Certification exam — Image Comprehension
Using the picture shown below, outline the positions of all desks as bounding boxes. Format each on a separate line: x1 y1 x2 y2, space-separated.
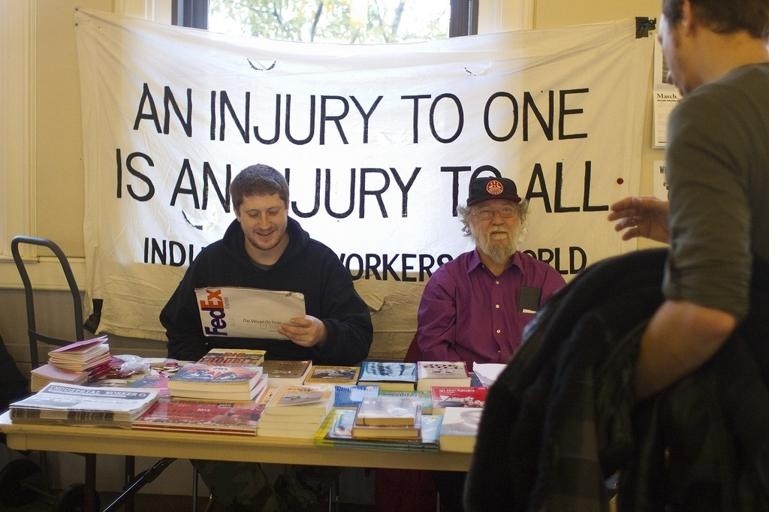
0 409 474 512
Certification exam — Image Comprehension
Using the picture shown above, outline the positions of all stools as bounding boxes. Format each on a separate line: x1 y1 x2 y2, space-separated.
193 467 340 512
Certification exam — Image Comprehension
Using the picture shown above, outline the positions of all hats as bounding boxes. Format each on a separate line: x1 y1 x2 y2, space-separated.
467 176 522 207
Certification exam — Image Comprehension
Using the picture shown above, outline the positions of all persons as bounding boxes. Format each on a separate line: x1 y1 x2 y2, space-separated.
608 0 766 512
158 165 373 511
407 174 571 511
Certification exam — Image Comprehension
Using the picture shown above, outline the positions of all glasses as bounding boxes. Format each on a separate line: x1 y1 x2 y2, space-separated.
477 207 516 221
